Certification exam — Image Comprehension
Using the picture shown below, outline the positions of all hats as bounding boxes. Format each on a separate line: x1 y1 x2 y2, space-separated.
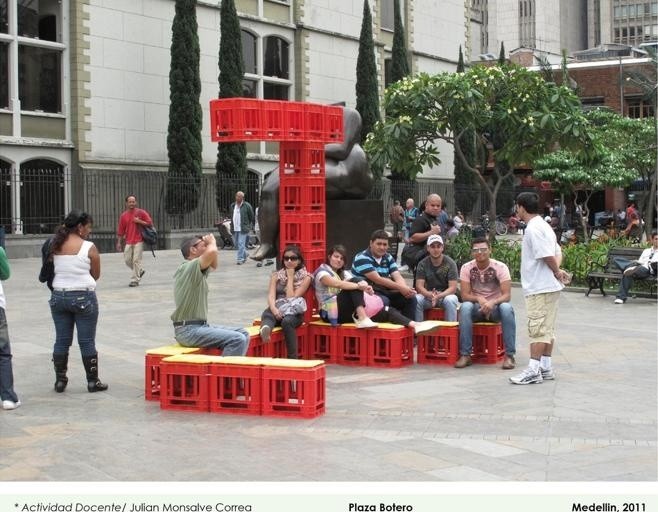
426 233 444 247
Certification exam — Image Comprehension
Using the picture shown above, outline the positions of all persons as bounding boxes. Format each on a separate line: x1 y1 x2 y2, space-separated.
352 230 418 321
543 199 566 227
259 246 311 359
508 192 570 384
390 201 405 239
613 232 658 304
509 205 526 233
39 210 108 392
313 245 441 335
452 211 464 227
117 196 153 288
255 206 274 268
599 204 647 240
231 191 254 265
171 234 250 356
455 238 515 369
404 198 418 240
410 194 441 292
435 203 450 225
415 235 458 322
442 220 459 242
0 247 22 411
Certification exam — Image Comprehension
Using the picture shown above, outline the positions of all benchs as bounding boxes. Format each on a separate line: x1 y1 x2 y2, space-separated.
585 246 658 301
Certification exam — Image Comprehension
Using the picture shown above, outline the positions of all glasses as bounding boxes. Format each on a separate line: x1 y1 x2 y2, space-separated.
282 255 301 261
471 246 490 254
649 253 655 259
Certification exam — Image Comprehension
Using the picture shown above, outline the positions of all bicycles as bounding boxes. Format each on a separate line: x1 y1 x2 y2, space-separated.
215 219 258 249
486 215 508 234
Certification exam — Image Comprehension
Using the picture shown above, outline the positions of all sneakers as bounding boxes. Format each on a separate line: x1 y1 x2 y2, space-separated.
414 319 441 338
355 316 380 330
613 296 626 306
237 259 247 265
260 324 272 344
256 262 263 267
244 253 249 258
128 280 139 288
622 262 641 278
1 398 22 409
542 368 557 382
264 261 275 266
139 267 145 278
508 370 545 386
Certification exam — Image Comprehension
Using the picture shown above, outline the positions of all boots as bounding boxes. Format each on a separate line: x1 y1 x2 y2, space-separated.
53 352 69 391
80 353 107 393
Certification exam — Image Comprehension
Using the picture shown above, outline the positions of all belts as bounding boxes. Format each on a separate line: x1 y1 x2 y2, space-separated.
173 320 207 326
54 287 96 291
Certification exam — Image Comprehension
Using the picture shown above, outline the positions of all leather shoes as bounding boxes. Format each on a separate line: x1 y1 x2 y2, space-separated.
502 355 515 370
453 353 475 367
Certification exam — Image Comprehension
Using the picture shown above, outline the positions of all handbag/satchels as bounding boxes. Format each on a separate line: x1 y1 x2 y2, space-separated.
401 215 435 275
275 297 308 318
138 208 159 246
